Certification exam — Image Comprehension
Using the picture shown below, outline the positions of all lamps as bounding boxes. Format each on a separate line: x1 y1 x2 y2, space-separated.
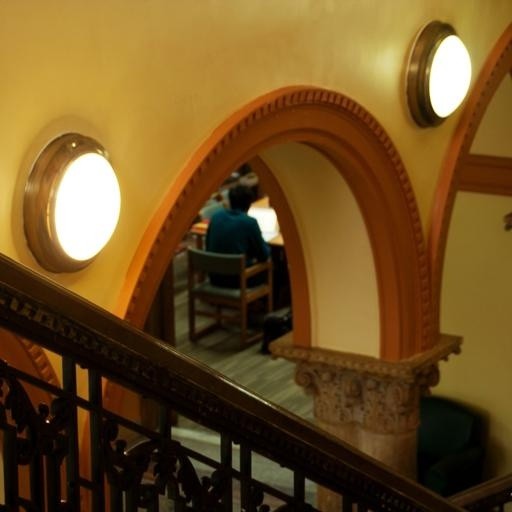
403 19 471 129
23 131 123 276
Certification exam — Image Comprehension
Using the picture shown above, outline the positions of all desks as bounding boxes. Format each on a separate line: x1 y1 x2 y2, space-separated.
191 195 287 251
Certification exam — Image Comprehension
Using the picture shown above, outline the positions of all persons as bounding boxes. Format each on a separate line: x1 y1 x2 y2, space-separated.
205 185 286 315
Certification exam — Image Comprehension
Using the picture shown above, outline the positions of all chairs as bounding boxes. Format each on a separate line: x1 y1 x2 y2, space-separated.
185 245 275 351
